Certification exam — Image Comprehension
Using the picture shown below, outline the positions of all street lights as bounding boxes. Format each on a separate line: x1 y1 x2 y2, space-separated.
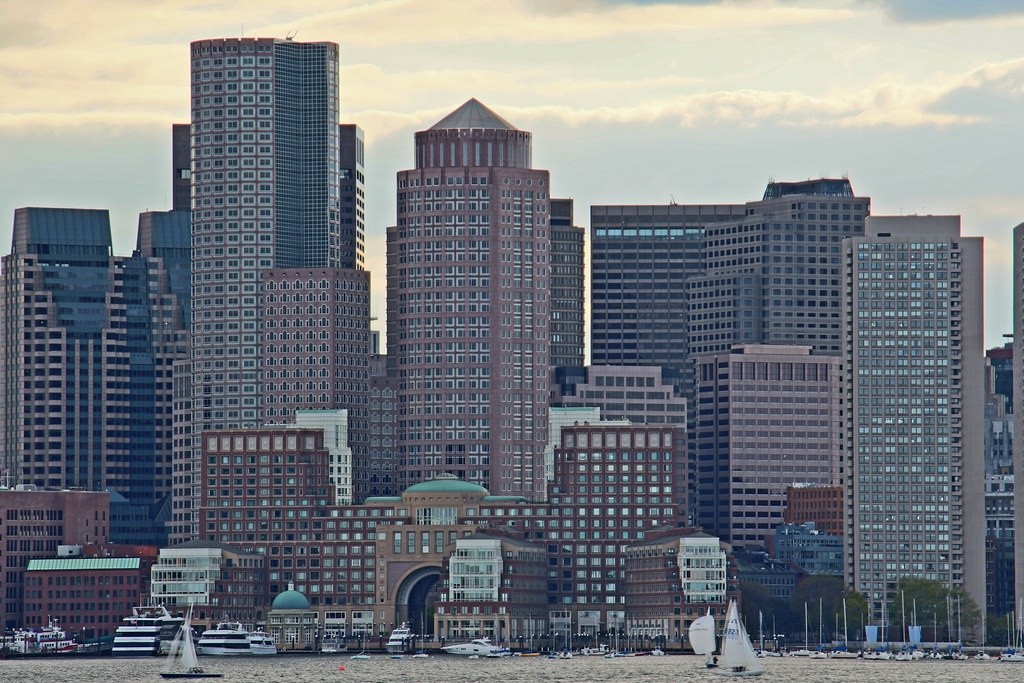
83 627 86 650
12 626 15 643
531 633 785 654
92 625 94 648
292 634 429 650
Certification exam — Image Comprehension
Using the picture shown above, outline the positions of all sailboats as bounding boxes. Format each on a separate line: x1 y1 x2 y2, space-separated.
912 595 1024 662
159 602 224 678
689 605 719 668
831 598 859 658
809 598 828 659
789 602 819 656
863 605 894 659
712 597 766 677
894 590 913 661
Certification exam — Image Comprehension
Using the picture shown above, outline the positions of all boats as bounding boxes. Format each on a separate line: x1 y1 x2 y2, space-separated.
196 622 277 655
490 647 513 656
386 622 415 653
112 606 170 656
350 655 371 660
412 654 428 658
441 639 500 656
520 650 540 656
560 651 572 658
584 648 605 655
